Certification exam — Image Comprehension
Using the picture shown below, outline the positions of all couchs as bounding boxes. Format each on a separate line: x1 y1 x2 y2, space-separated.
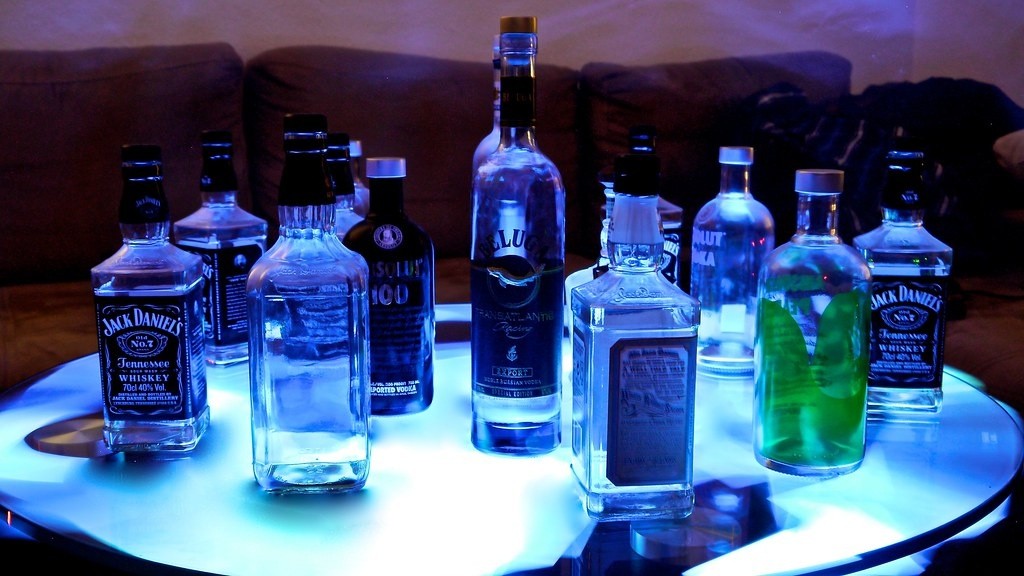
1 34 1023 411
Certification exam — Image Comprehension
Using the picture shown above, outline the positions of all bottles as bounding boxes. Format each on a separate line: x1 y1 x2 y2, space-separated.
612 131 686 292
91 142 212 454
246 112 372 495
347 138 371 218
472 33 510 199
691 146 775 377
320 132 366 248
571 149 701 522
853 150 954 417
467 15 563 457
171 129 269 368
341 156 434 419
563 180 631 450
753 168 868 477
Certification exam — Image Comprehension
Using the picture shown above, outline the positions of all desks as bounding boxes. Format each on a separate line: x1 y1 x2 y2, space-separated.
0 303 1023 575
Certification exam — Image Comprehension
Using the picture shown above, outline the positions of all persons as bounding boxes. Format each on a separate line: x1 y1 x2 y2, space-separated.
682 76 1023 311
